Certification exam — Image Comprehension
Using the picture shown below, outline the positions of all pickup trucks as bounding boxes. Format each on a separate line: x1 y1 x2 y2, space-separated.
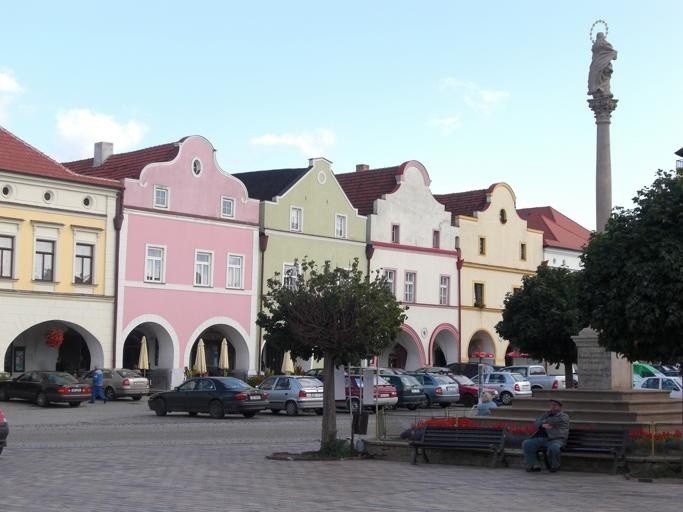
496 365 566 390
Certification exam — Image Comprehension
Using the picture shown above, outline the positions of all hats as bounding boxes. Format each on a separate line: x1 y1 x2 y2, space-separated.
549 400 562 406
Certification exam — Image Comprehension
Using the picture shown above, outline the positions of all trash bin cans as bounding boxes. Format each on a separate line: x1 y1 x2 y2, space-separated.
0 372 11 402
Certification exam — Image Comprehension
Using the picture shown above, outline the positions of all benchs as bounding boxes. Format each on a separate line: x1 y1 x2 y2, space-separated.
409 425 510 468
528 428 630 475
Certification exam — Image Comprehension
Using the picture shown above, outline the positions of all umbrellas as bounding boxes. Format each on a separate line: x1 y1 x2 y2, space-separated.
471 351 495 364
194 338 207 377
137 336 149 377
505 351 530 365
281 350 294 375
218 338 229 376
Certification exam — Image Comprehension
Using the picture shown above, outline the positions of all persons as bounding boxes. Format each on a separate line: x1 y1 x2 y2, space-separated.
523 399 570 473
89 365 106 403
133 365 142 374
475 393 498 416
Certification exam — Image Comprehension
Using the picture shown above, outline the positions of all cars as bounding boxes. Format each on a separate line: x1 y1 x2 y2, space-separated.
0 368 149 407
632 363 682 399
0 407 9 455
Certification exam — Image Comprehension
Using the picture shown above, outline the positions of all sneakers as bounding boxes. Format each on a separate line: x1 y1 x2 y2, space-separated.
550 466 560 472
526 467 541 472
